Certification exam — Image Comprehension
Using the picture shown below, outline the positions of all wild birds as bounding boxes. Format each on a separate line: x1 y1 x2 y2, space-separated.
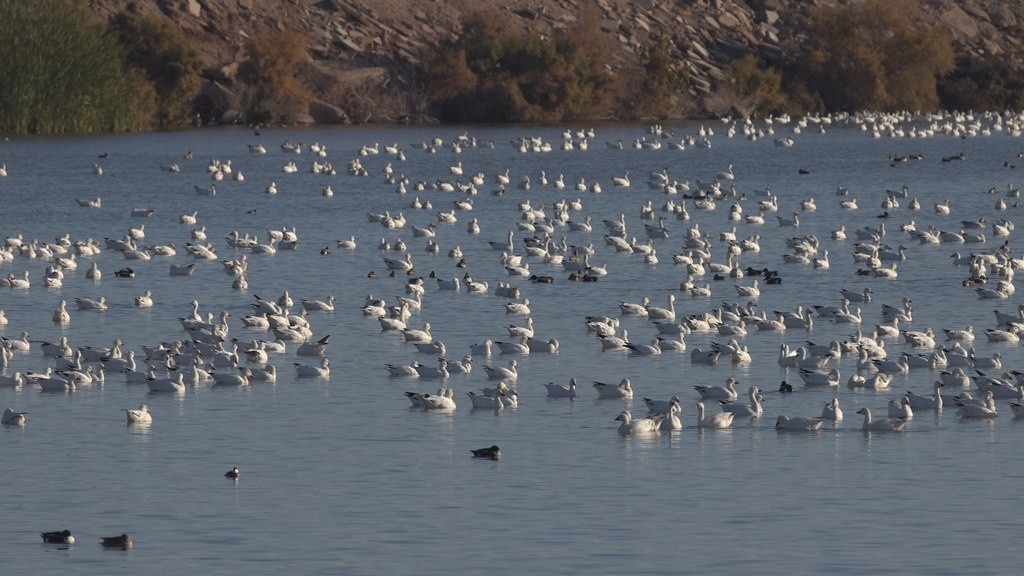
40 530 75 544
224 467 240 478
722 109 1022 146
587 121 1024 443
0 125 612 432
469 445 501 456
101 534 133 550
